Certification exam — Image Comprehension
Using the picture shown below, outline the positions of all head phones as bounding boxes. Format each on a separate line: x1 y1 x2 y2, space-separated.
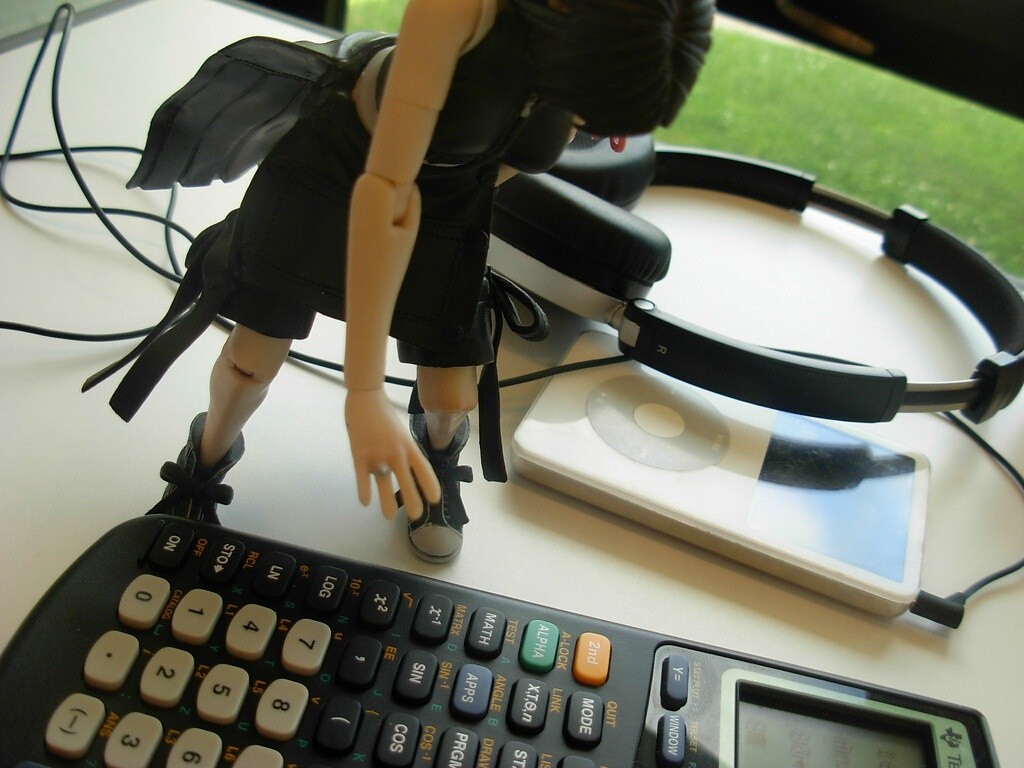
488 128 1024 425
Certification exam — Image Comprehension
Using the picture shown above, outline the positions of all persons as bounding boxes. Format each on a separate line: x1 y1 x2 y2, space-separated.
146 0 715 565
